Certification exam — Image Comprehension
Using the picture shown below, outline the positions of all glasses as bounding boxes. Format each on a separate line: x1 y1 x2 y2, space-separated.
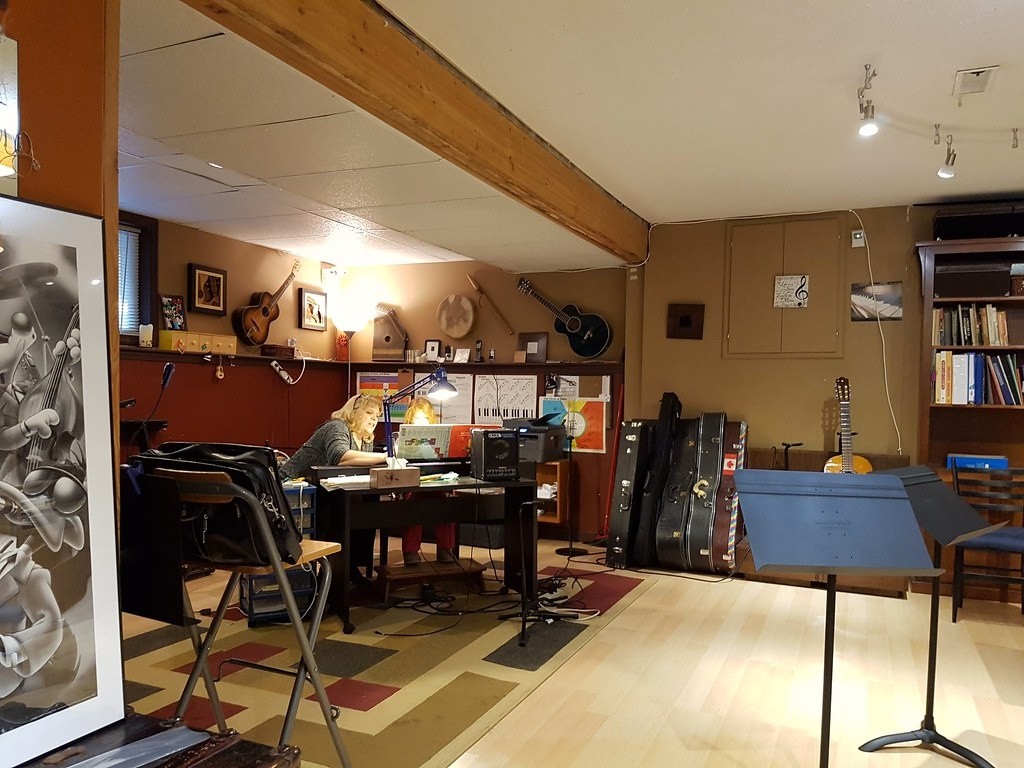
354 393 367 405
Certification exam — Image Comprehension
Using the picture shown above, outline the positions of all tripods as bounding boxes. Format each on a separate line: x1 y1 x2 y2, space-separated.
497 501 579 647
859 464 996 768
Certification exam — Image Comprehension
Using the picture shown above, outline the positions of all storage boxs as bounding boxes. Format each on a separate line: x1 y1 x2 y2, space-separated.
261 344 294 359
158 330 237 355
371 468 420 488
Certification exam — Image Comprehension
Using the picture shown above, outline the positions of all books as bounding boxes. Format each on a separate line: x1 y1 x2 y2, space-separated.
397 424 502 459
932 303 1008 346
932 349 1024 406
947 453 1008 471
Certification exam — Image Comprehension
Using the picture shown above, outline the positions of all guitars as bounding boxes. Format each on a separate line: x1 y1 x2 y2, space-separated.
230 258 303 347
516 276 614 359
822 374 874 476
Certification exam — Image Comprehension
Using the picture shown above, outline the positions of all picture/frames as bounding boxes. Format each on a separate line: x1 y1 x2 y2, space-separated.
298 288 327 331
187 263 227 316
0 193 125 768
159 293 188 330
424 339 441 362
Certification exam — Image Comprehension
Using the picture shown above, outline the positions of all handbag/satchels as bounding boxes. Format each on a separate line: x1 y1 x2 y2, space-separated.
142 441 303 565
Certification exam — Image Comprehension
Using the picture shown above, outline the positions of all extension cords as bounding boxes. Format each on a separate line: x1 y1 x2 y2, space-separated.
271 360 293 385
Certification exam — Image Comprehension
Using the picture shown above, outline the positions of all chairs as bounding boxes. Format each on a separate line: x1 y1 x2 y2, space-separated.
950 457 1024 623
133 466 355 768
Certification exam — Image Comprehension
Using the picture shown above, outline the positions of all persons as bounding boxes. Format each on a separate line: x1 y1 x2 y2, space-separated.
393 397 455 564
278 392 388 588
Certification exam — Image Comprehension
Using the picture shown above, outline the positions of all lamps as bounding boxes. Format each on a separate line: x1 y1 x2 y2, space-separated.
382 364 458 457
330 298 367 400
860 100 879 137
937 135 956 178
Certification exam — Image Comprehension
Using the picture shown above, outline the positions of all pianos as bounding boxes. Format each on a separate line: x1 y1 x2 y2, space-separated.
309 460 541 633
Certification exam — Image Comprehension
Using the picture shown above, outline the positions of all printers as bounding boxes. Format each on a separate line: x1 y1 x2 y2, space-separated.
503 413 566 464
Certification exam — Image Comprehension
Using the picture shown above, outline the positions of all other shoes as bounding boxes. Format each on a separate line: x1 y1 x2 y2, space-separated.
404 551 420 565
350 568 373 587
437 548 454 562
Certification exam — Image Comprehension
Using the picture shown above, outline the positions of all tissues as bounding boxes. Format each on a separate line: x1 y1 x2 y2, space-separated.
370 457 420 489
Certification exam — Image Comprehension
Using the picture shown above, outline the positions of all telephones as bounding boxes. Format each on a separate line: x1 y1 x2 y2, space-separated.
473 339 484 363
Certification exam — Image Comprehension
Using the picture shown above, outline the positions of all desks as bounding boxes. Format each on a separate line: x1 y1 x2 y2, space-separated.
302 472 538 635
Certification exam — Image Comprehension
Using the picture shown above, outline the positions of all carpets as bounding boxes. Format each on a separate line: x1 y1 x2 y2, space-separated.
123 549 659 768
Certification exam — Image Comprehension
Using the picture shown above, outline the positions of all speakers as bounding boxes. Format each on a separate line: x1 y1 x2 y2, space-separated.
472 428 520 480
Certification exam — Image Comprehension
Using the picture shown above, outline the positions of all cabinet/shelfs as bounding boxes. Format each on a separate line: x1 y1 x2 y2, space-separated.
910 238 1024 602
723 213 843 358
239 483 317 628
